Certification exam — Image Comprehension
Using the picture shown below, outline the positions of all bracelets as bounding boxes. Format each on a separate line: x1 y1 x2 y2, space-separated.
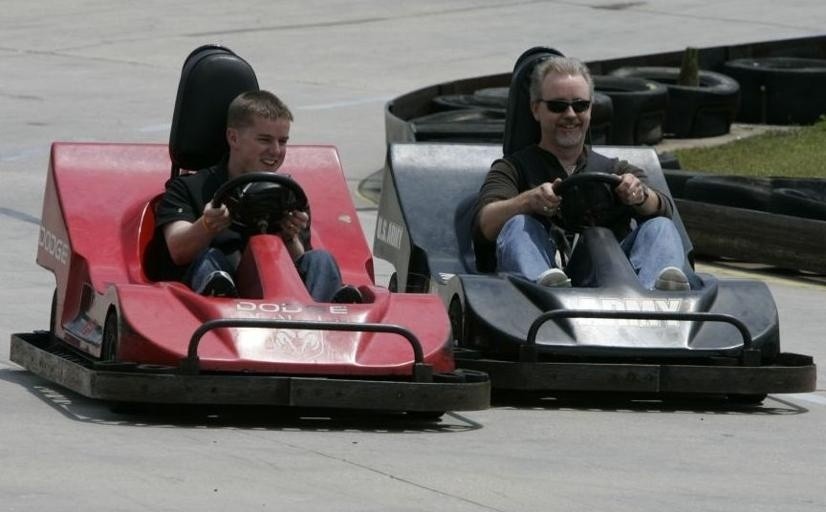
634 184 650 206
200 215 215 236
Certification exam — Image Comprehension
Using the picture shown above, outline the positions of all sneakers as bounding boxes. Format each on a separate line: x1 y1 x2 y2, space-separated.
655 267 690 292
198 271 236 297
537 268 571 287
331 286 363 303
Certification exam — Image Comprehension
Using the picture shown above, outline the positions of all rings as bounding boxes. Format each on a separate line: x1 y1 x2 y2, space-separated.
542 205 549 215
286 224 294 229
630 191 638 197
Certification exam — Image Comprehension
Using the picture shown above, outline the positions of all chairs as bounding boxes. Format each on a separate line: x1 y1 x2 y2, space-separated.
454 45 567 273
137 45 261 280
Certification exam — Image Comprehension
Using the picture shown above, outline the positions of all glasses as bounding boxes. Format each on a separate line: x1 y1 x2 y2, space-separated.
537 99 591 113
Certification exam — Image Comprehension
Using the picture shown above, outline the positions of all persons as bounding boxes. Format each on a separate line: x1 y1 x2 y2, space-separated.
469 54 704 296
141 88 367 306
